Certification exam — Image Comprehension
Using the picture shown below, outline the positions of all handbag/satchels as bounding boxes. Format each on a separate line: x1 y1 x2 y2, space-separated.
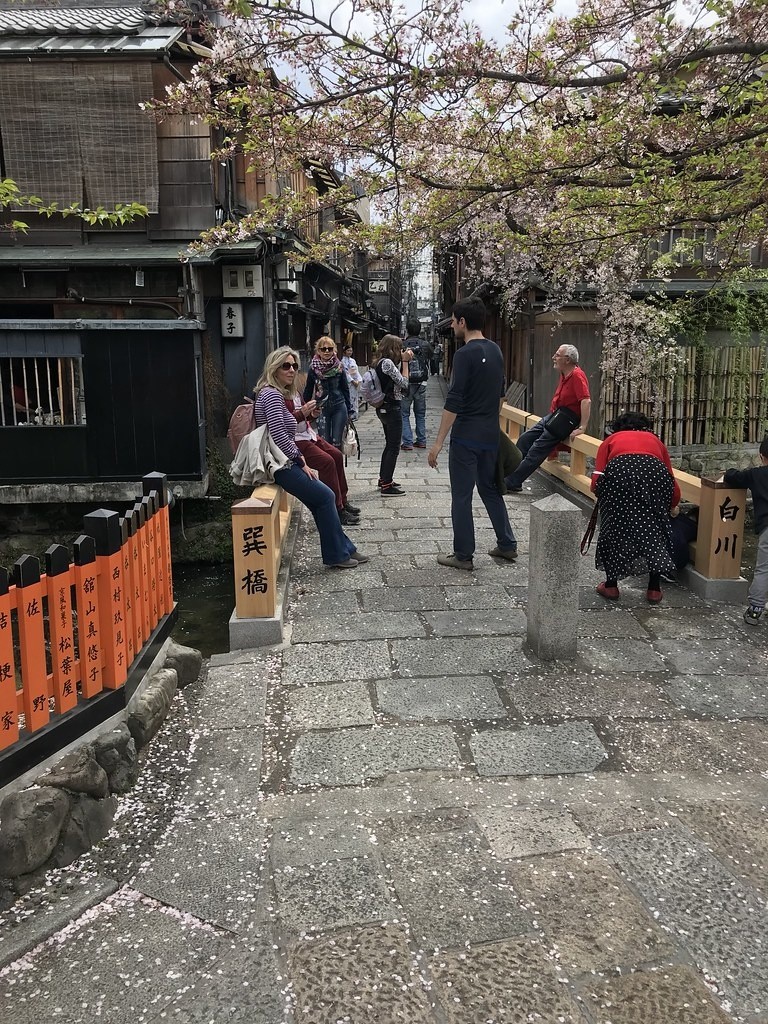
544 407 580 440
341 418 359 456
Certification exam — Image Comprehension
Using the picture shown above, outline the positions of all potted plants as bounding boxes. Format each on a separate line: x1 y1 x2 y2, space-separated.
371 337 378 352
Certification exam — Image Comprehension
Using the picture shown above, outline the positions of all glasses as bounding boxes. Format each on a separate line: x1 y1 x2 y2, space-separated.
318 346 334 352
554 353 569 358
277 362 299 371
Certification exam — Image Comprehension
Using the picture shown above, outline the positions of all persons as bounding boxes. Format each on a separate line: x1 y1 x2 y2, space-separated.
400 319 435 450
723 435 768 626
428 296 518 568
497 344 591 494
400 335 443 376
590 412 681 603
371 334 414 497
254 345 369 568
341 345 363 422
303 337 352 451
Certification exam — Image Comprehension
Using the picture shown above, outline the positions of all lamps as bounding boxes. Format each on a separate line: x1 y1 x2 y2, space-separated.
365 298 373 308
384 314 389 321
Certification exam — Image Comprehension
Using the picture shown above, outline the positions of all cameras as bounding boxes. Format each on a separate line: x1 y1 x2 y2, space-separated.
403 345 421 355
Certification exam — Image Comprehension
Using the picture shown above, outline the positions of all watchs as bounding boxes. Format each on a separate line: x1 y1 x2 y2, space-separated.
579 426 586 431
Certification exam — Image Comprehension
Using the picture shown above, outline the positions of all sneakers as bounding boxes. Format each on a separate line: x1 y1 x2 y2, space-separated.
378 478 402 487
660 571 676 582
596 582 620 599
437 553 474 569
338 501 361 525
328 551 369 568
381 486 406 496
647 589 663 603
743 606 763 625
487 546 519 559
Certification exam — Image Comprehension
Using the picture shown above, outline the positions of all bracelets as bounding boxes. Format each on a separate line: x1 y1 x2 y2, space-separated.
402 361 408 362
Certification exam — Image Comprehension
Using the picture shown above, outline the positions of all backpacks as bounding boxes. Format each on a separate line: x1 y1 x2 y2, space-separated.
359 358 386 408
403 340 430 382
226 403 257 457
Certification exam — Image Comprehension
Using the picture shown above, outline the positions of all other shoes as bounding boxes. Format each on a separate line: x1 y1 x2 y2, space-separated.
503 480 523 492
401 443 414 450
413 441 427 448
